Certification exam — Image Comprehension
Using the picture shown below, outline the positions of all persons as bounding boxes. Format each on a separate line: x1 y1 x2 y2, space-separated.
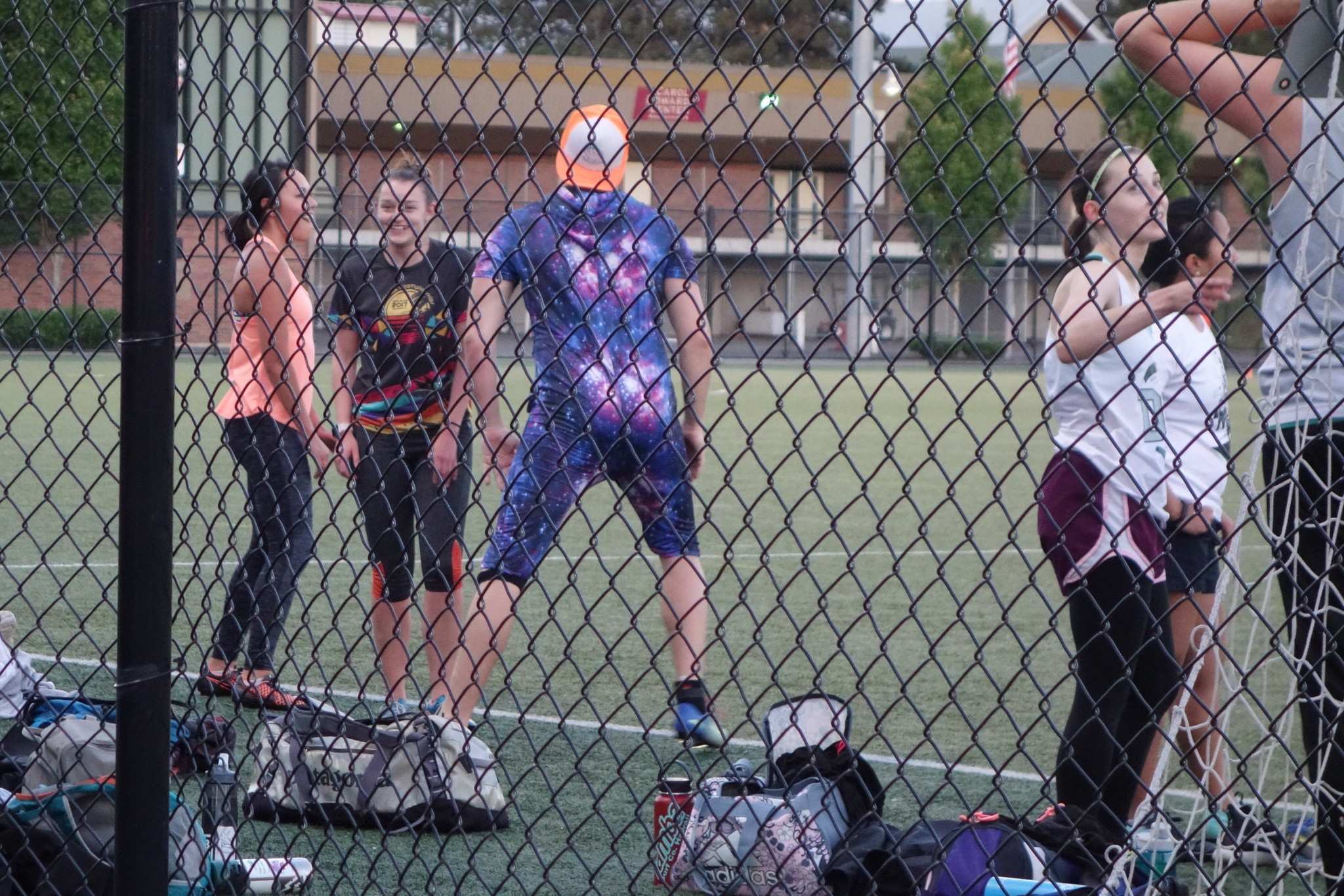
1110 0 1343 896
325 160 480 732
442 102 734 752
1037 139 1233 896
1121 195 1234 845
196 161 342 711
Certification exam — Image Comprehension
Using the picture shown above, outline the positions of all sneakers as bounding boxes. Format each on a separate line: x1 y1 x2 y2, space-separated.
672 702 730 749
1125 811 1217 860
196 664 246 695
1211 802 1281 866
1276 813 1324 870
1201 803 1231 843
232 675 308 710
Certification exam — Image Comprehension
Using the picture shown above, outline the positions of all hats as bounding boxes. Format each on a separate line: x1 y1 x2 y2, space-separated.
555 104 631 193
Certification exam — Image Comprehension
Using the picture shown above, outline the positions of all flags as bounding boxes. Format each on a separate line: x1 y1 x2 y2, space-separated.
1004 4 1023 86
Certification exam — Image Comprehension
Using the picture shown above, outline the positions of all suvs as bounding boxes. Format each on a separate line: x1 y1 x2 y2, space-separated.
815 300 896 342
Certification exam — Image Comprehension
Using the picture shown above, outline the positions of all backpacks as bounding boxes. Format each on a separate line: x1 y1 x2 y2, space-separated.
21 711 116 793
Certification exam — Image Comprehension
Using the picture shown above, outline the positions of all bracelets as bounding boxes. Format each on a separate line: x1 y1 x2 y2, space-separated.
336 423 355 434
1171 497 1187 524
450 421 461 429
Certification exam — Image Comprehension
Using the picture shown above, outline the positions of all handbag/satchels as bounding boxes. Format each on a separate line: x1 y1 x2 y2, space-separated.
668 773 849 896
241 700 511 834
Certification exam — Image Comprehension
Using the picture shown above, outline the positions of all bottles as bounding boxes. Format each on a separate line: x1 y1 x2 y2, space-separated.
652 759 698 884
209 857 314 895
201 752 241 862
1133 819 1178 883
